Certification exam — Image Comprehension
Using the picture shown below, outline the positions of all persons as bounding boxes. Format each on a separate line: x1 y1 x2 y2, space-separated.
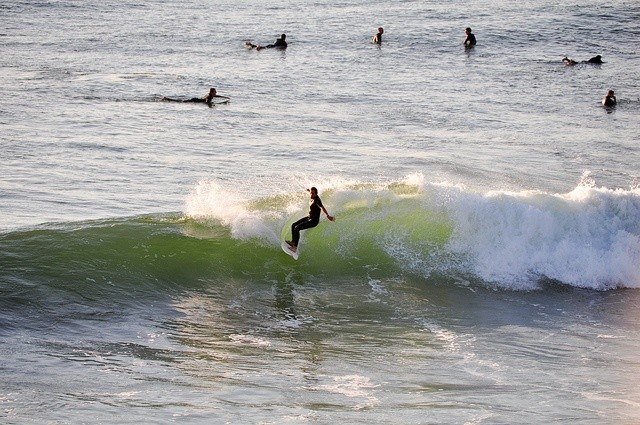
372 29 384 46
285 185 337 253
463 27 477 51
244 33 289 52
160 88 233 108
601 89 617 113
562 55 605 65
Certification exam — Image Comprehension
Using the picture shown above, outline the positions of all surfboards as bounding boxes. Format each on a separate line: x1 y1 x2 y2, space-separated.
281 222 300 260
216 98 229 104
